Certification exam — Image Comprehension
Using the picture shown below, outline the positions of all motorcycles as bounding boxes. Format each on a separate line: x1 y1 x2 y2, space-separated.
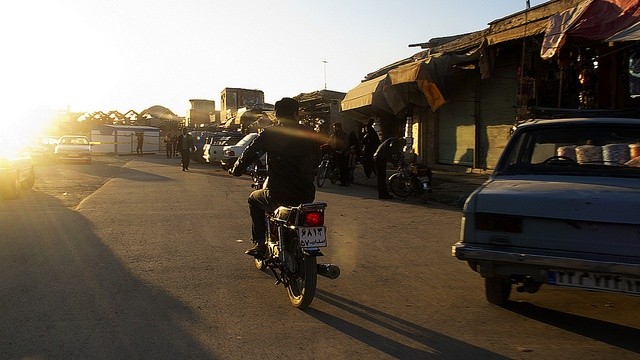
230 165 340 311
317 146 354 187
389 150 433 204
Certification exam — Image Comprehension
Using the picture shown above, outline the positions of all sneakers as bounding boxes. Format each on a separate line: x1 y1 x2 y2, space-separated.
244 244 269 261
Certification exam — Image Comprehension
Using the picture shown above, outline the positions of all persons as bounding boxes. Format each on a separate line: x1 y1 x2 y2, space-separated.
176 133 183 152
135 134 144 156
319 121 351 186
360 123 379 179
228 97 322 256
171 134 180 157
372 137 408 199
164 132 175 159
175 128 195 171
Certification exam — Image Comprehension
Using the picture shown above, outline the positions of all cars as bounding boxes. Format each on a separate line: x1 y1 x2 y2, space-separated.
187 131 223 166
450 118 638 306
223 131 266 177
224 132 243 146
54 136 92 165
